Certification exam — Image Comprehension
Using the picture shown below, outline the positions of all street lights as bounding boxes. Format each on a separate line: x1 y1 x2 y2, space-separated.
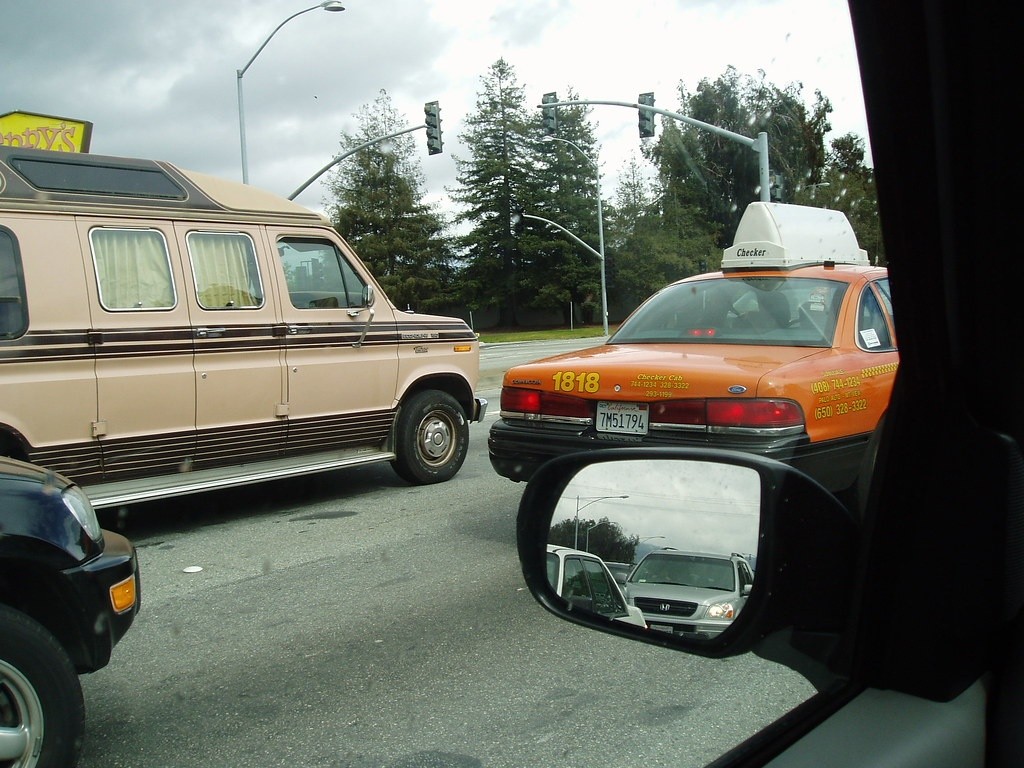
236 0 346 187
586 522 619 551
520 136 610 337
634 535 666 565
574 494 629 552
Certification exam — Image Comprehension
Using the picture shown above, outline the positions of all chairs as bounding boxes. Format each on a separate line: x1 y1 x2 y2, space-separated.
798 300 828 328
733 311 770 331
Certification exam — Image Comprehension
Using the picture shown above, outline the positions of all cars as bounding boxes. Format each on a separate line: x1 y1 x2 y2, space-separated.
624 547 755 640
488 200 900 505
547 543 648 628
0 455 142 768
605 562 639 605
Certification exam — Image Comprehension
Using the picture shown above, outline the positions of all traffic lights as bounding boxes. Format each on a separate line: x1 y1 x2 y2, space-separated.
638 91 657 138
542 92 559 134
424 101 445 156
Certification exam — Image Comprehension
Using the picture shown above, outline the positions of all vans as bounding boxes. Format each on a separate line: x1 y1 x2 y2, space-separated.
0 145 489 511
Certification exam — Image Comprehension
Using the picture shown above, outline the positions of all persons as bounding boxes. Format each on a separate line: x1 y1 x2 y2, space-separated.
757 291 792 327
